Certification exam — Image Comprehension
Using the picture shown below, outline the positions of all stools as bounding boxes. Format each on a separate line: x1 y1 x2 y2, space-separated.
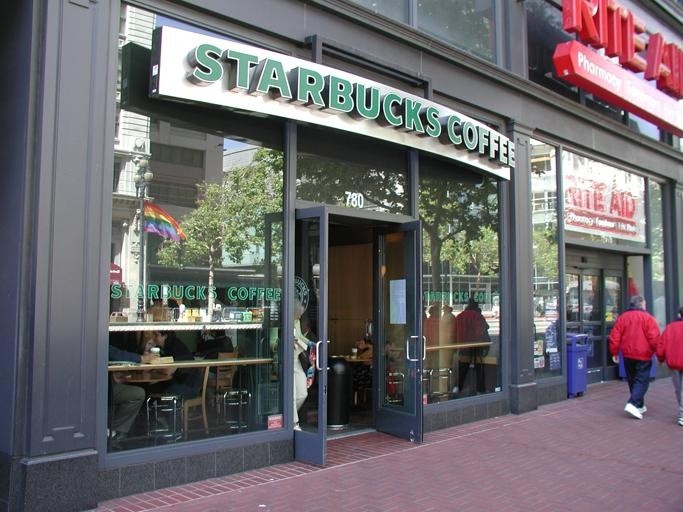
386 369 406 408
146 391 184 446
420 368 453 406
216 388 250 433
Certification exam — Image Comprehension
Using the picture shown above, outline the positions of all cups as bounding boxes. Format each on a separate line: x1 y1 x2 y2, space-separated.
150 347 160 358
351 347 358 359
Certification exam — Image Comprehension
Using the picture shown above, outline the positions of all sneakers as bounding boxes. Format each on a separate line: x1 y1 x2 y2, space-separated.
624 403 646 419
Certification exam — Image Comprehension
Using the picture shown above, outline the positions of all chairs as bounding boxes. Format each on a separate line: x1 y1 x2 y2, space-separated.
209 345 242 413
178 362 212 433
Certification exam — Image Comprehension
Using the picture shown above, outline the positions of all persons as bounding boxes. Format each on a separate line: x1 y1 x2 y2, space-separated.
196 330 234 380
273 296 375 433
422 299 492 402
145 330 202 442
657 306 683 426
108 344 159 451
608 296 660 420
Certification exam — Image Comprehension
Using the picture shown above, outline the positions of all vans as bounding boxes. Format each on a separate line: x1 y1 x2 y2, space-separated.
566 279 620 321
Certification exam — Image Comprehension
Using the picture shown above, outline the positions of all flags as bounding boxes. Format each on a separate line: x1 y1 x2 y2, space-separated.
142 202 185 241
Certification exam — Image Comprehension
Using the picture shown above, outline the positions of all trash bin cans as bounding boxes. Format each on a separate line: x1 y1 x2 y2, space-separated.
618 350 658 381
566 333 592 399
327 357 352 431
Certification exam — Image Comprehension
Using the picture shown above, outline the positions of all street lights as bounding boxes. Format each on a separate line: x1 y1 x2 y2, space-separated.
130 158 155 322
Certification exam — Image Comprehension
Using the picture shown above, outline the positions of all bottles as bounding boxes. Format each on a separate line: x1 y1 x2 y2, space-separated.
177 303 186 322
